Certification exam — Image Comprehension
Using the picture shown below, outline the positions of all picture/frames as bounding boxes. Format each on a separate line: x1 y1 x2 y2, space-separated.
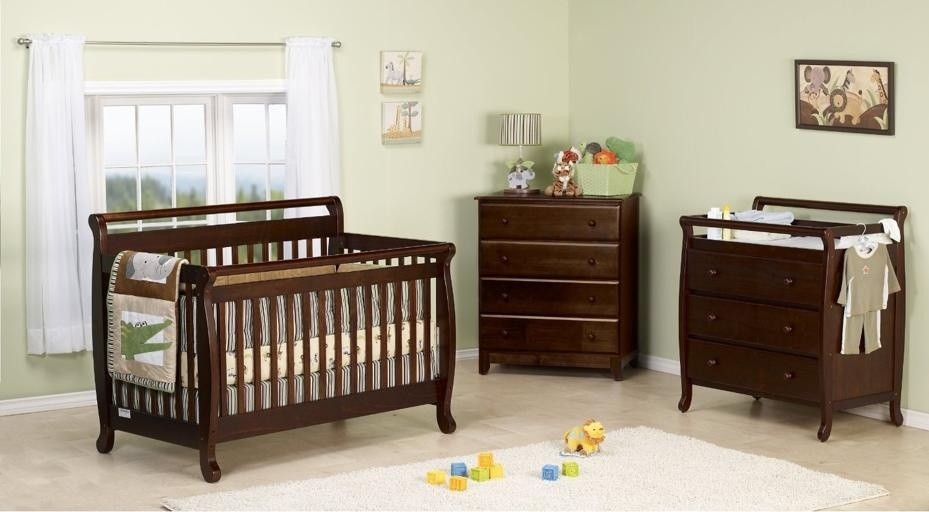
379 48 426 148
791 58 898 138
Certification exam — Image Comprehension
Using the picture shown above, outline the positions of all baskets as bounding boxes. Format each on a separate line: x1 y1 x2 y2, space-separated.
575 163 639 197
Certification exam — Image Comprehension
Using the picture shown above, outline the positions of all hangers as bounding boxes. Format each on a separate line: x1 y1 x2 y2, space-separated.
850 219 877 254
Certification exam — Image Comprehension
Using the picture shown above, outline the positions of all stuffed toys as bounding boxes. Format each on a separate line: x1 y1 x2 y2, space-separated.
545 136 637 197
563 416 606 455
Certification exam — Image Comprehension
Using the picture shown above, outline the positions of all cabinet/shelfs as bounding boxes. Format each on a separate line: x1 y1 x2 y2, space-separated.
471 187 646 384
676 192 913 445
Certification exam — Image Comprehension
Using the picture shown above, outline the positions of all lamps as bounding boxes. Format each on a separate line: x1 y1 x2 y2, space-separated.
498 112 544 196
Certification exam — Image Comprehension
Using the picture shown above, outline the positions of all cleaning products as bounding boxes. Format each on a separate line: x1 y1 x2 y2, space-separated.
706 208 722 240
723 204 732 240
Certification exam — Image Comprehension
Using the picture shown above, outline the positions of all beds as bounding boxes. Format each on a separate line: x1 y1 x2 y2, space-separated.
84 196 462 485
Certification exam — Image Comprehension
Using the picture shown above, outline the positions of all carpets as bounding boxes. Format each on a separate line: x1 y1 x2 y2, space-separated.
153 424 899 508
